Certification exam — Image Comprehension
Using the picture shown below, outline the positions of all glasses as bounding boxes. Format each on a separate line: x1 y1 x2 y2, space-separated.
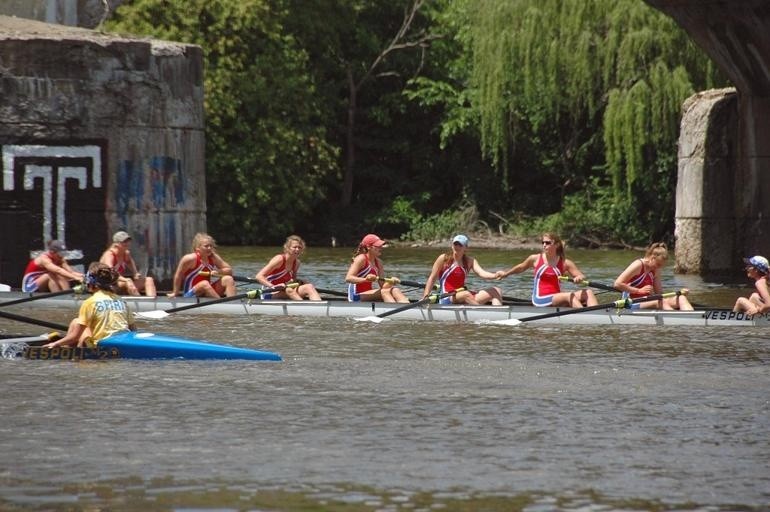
542 241 553 244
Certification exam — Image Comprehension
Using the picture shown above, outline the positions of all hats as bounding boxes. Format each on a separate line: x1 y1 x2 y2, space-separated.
453 235 468 246
362 234 385 248
744 256 769 273
50 240 68 257
112 231 130 244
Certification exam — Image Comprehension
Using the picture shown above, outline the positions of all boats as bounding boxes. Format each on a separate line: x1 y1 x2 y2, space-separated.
0 287 770 328
0 331 284 362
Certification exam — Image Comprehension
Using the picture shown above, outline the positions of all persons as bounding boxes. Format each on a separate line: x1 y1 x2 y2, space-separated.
255 235 322 301
99 230 157 296
167 232 236 298
733 255 770 316
613 242 694 311
424 234 503 306
345 234 410 303
22 240 84 293
496 232 598 308
41 261 137 349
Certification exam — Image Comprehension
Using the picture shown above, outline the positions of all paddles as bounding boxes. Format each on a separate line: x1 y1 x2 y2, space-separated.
0 283 22 291
137 283 299 319
354 286 467 324
491 289 687 327
